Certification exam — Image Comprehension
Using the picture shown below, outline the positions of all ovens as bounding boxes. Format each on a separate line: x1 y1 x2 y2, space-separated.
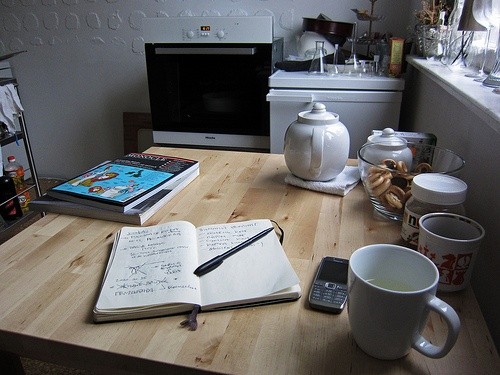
141 14 284 151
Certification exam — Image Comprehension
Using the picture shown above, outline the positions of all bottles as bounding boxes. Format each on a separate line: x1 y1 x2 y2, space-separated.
308 41 329 76
0 175 23 226
4 155 32 211
401 173 468 250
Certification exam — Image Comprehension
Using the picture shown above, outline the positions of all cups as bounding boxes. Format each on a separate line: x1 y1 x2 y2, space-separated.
347 244 460 361
418 213 486 293
305 48 327 72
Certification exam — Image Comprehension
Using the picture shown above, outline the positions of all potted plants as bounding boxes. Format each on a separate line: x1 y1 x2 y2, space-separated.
407 0 453 62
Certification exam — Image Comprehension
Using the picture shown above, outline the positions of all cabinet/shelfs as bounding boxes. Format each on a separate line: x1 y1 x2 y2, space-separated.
0 60 46 237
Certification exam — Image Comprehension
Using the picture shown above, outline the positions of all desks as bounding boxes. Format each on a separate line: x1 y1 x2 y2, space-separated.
0 147 500 375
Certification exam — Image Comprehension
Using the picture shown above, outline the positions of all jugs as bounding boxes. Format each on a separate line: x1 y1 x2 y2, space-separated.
283 103 350 183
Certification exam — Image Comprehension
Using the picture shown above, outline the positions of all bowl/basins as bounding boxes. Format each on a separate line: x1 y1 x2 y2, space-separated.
302 17 353 48
357 141 466 222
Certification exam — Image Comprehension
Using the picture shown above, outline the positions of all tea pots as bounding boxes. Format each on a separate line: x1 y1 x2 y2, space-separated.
295 30 334 57
364 128 413 183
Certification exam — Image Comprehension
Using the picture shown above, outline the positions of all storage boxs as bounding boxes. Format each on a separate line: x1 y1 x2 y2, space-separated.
386 37 403 77
372 130 437 168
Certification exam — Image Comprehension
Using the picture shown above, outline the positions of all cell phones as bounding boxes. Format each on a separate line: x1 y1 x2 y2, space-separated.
308 255 351 313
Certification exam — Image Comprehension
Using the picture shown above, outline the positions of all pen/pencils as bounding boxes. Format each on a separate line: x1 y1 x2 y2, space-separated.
194 227 274 277
